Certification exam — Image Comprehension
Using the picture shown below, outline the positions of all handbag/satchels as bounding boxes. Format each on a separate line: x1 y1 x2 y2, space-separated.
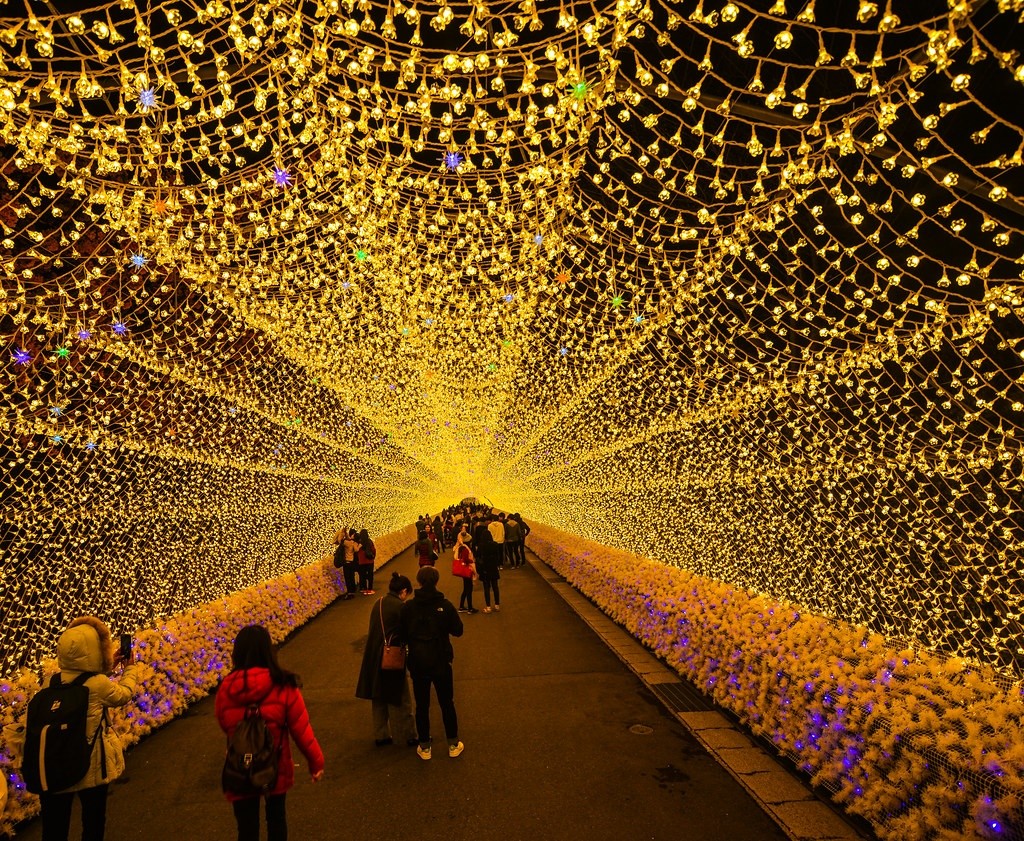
366 550 375 560
382 632 407 669
452 559 475 579
428 550 439 560
333 539 346 568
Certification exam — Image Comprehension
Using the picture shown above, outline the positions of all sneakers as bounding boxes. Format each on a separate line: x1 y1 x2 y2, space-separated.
448 741 464 757
417 745 432 760
468 608 478 614
458 607 468 612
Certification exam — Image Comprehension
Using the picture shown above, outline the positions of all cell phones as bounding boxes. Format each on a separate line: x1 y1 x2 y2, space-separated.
120 634 132 659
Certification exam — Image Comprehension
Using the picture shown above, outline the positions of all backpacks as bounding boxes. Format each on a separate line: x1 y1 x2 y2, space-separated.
221 685 288 798
22 671 111 795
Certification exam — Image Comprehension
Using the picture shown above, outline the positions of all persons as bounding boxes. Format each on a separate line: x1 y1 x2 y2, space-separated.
400 566 465 760
38 616 137 841
355 572 433 744
215 624 326 840
414 498 530 615
343 528 376 597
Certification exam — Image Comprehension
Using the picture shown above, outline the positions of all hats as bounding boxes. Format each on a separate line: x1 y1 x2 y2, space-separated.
458 531 472 542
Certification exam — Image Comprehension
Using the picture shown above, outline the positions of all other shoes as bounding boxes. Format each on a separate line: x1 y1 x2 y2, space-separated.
364 590 375 595
406 736 433 746
482 607 492 613
359 590 363 593
344 593 356 600
376 737 392 746
497 562 526 570
495 605 499 611
